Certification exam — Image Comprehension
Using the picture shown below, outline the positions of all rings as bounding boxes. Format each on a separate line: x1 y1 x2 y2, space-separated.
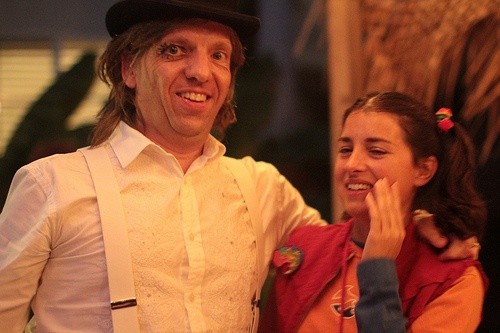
470 242 482 251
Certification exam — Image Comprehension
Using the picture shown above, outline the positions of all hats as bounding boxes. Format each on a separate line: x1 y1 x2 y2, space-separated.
105 0 262 45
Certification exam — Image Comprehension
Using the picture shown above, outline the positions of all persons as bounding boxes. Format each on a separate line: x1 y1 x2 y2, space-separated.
257 91 489 333
0 0 480 333
